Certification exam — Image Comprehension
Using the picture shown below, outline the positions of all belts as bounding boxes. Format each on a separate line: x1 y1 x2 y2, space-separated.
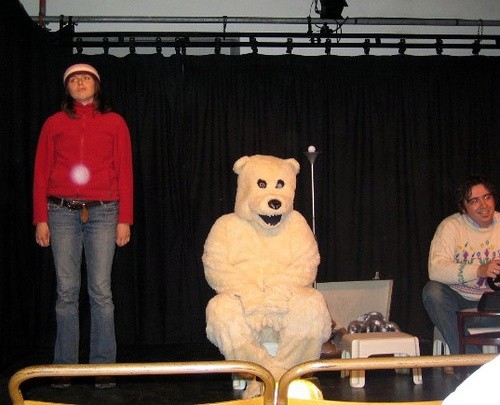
47 196 112 210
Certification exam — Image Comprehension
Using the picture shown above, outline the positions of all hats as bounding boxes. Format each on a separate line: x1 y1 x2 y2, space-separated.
63 63 101 83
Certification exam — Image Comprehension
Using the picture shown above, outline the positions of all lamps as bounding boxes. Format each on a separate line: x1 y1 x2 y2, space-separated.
303 146 321 289
313 0 349 19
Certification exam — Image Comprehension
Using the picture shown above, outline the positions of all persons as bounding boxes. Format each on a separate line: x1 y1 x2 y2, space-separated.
423 183 500 370
36 60 135 388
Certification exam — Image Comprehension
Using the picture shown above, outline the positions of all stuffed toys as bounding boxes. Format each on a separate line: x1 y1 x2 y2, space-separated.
201 152 331 398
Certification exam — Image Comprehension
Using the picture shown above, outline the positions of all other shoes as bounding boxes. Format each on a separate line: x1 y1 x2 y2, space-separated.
95 376 117 389
51 377 71 389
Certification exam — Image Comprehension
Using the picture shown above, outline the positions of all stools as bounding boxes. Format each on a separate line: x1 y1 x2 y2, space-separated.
341 332 422 387
433 307 500 369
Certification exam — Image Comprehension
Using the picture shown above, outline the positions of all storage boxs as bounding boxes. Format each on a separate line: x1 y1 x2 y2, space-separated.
315 277 396 379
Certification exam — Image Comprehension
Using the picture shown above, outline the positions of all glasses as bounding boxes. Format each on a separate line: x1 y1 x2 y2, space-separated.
467 195 491 205
70 76 91 84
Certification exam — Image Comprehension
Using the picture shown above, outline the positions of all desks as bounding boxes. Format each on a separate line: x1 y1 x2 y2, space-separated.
457 311 500 381
